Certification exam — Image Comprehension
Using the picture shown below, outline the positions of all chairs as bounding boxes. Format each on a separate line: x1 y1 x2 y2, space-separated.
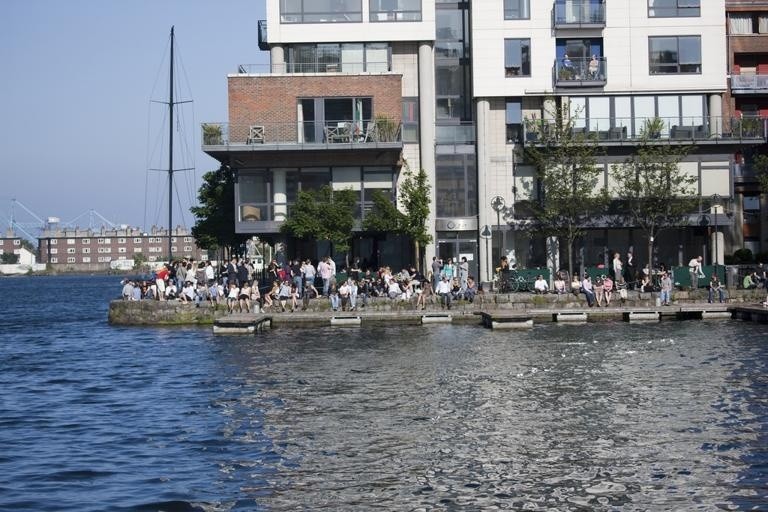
246 124 266 146
319 122 378 144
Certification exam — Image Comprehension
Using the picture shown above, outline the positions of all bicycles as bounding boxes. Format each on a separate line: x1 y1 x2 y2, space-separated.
501 271 539 293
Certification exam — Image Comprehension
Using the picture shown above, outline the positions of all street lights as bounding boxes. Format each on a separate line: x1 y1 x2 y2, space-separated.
491 196 509 270
708 193 722 282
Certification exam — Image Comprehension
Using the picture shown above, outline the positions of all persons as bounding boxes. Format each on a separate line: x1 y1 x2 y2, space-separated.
562 54 580 81
589 54 598 75
